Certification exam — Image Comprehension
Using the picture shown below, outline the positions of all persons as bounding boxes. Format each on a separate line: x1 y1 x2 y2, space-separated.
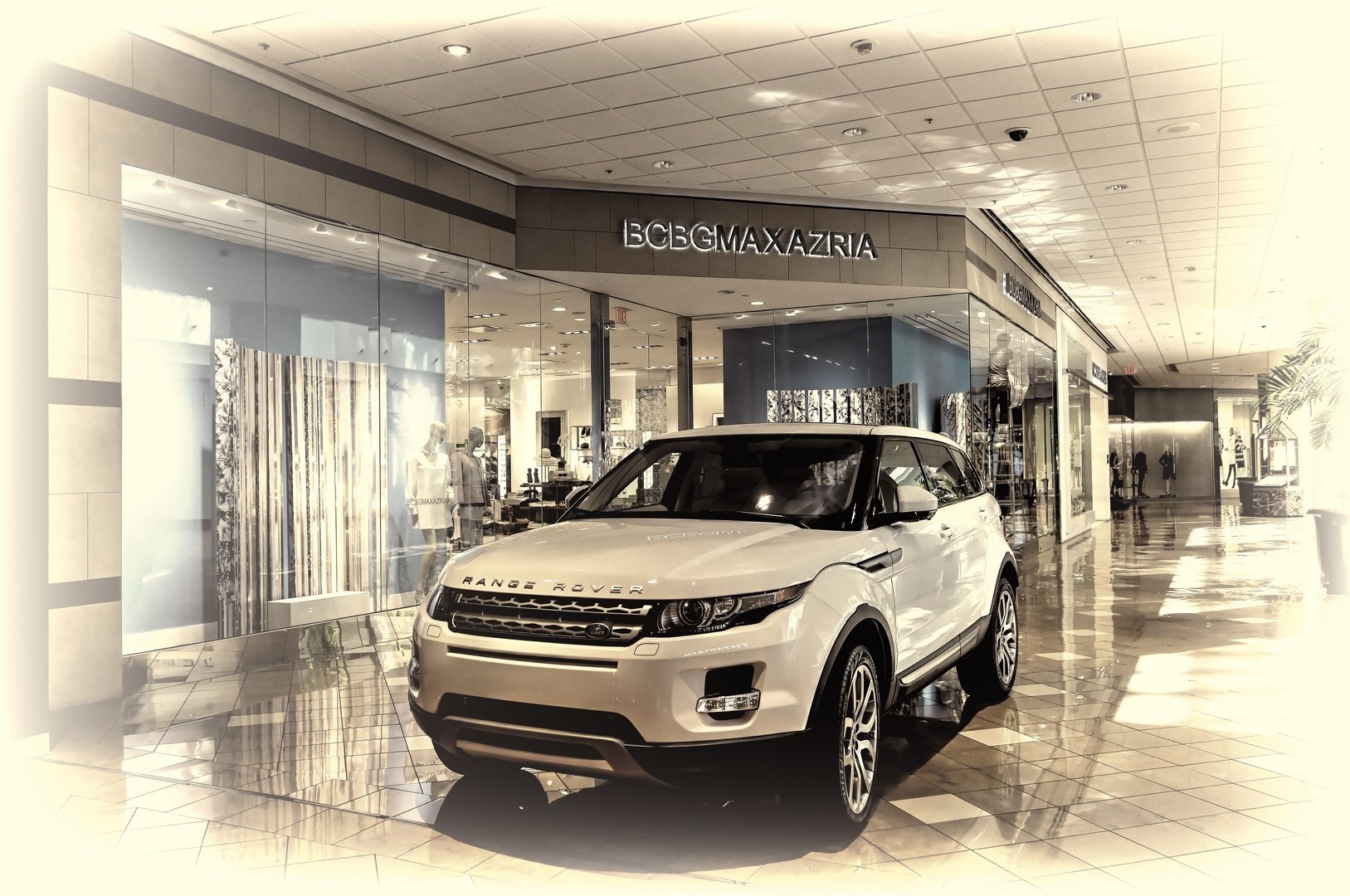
479 444 499 498
451 426 490 548
1233 428 1248 486
1221 426 1238 488
1134 446 1148 497
410 423 459 601
988 332 1012 439
1158 445 1176 495
1109 445 1120 498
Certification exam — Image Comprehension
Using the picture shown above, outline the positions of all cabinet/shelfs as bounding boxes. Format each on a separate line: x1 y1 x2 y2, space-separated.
523 479 593 531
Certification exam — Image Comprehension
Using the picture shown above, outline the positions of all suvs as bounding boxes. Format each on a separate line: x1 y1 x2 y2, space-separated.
404 421 1022 842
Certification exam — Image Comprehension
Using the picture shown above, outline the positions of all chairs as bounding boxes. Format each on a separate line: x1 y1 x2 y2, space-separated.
690 467 759 513
